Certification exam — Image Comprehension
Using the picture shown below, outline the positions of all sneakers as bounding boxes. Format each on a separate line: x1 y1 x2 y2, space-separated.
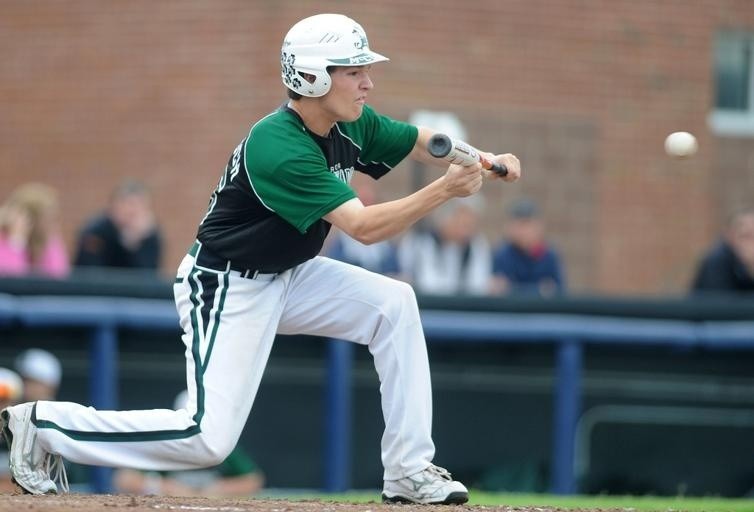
381 467 469 504
1 399 57 494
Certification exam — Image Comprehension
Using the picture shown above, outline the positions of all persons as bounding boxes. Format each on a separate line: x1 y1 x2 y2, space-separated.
0 10 523 505
392 186 513 299
686 204 754 295
75 183 164 270
494 199 569 298
0 347 95 493
109 387 264 498
0 366 26 480
320 173 415 290
0 181 72 281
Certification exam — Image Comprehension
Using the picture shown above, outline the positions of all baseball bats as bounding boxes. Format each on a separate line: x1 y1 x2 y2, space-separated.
427 134 506 174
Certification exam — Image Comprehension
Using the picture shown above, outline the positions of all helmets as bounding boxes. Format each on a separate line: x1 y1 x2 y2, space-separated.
280 14 390 98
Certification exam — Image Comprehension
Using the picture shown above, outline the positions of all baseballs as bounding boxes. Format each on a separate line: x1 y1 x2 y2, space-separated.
664 131 698 159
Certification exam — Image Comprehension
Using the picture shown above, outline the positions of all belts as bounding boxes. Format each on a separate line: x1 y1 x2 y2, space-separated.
188 242 277 275
